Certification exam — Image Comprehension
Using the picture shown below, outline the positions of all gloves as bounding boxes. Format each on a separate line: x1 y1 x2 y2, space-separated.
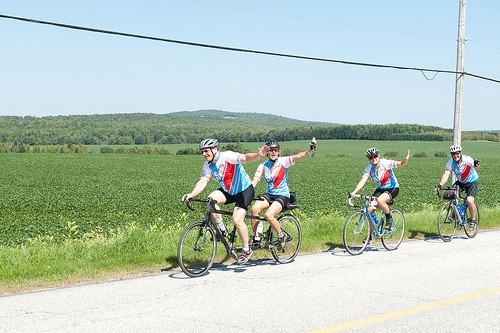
310 137 318 152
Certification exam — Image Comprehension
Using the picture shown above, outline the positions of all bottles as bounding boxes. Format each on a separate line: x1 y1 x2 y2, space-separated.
457 206 462 216
370 210 379 224
461 205 465 217
216 219 229 237
254 221 263 242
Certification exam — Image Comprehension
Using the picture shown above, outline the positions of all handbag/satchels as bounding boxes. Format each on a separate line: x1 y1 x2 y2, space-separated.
284 192 297 210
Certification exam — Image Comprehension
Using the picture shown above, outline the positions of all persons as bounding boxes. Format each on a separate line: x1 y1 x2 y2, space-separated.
182 138 270 264
248 137 317 251
348 147 410 244
434 144 481 231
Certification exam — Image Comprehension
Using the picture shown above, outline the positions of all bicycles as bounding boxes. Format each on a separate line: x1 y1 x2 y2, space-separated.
342 191 406 256
433 183 480 242
176 189 302 278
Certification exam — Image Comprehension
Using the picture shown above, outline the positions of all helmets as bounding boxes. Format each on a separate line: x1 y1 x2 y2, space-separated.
449 144 462 152
366 147 379 157
198 139 219 149
265 139 280 146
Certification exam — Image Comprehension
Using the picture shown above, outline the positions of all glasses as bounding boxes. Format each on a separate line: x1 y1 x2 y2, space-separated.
269 148 279 152
452 152 460 155
201 150 210 154
368 154 378 160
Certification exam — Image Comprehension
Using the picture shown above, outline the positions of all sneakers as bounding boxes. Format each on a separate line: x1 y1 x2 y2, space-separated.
277 233 287 251
469 219 477 230
364 236 372 244
238 248 253 265
385 216 393 229
211 229 228 241
